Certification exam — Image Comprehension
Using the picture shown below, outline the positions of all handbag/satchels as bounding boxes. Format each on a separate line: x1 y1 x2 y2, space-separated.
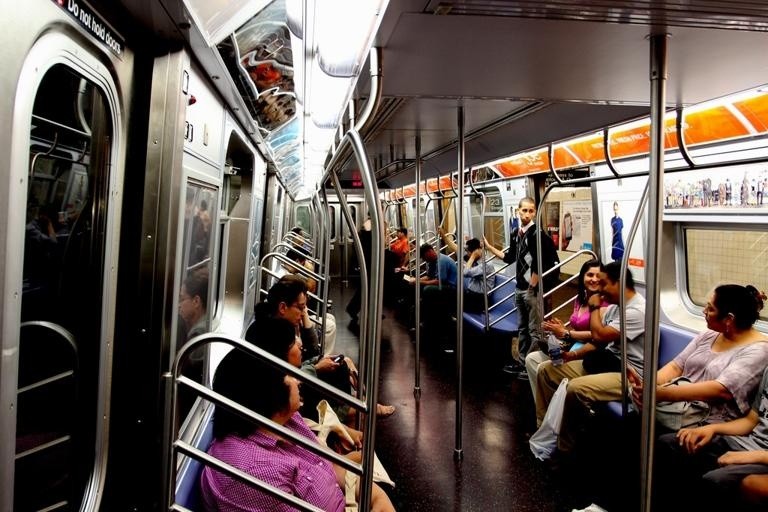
582 348 621 375
301 399 396 488
654 376 713 432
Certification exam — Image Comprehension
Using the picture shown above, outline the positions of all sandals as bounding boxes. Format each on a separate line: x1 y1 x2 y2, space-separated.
377 404 396 417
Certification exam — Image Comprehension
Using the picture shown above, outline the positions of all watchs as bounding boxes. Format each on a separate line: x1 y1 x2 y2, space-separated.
564 330 571 339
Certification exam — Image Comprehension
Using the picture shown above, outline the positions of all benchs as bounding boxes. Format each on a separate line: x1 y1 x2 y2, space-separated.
460 271 523 334
608 321 698 419
171 404 218 511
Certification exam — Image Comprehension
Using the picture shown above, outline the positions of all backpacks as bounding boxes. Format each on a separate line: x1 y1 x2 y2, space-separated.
547 236 561 280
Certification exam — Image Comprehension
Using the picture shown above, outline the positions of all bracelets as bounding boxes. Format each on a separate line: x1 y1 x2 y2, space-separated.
573 351 577 358
589 305 599 312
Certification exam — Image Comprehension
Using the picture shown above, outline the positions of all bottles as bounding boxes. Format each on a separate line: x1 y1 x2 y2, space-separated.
548 331 563 366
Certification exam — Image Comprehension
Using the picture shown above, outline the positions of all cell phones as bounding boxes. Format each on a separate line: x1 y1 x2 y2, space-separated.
333 354 344 362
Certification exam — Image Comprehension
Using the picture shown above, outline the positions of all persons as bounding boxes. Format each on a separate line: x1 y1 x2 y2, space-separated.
610 202 624 258
741 474 768 503
509 206 521 233
523 263 660 464
179 187 212 369
525 260 604 408
25 202 58 289
479 196 560 380
572 285 768 512
676 366 768 512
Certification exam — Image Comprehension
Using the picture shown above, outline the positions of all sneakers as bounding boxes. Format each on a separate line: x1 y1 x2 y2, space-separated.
518 370 529 381
345 307 359 321
571 503 609 512
503 362 523 374
